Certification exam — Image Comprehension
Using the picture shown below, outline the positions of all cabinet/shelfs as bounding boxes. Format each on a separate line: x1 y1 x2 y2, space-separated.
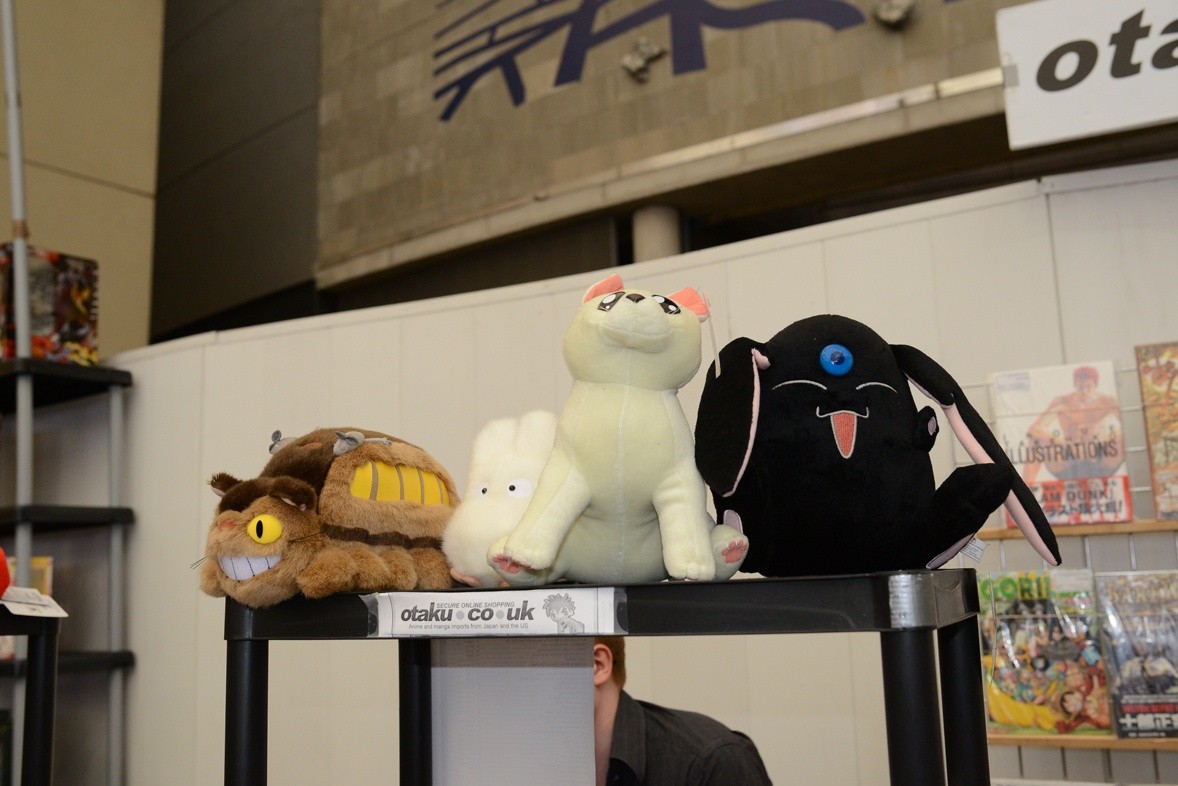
0 351 133 786
973 521 1178 753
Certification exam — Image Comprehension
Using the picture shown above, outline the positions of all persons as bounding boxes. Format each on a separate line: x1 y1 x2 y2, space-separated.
1020 362 1126 488
594 637 778 786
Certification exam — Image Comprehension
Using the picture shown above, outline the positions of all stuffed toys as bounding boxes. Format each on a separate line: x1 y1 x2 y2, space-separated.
694 312 1066 583
204 428 469 612
482 269 750 590
440 410 567 596
